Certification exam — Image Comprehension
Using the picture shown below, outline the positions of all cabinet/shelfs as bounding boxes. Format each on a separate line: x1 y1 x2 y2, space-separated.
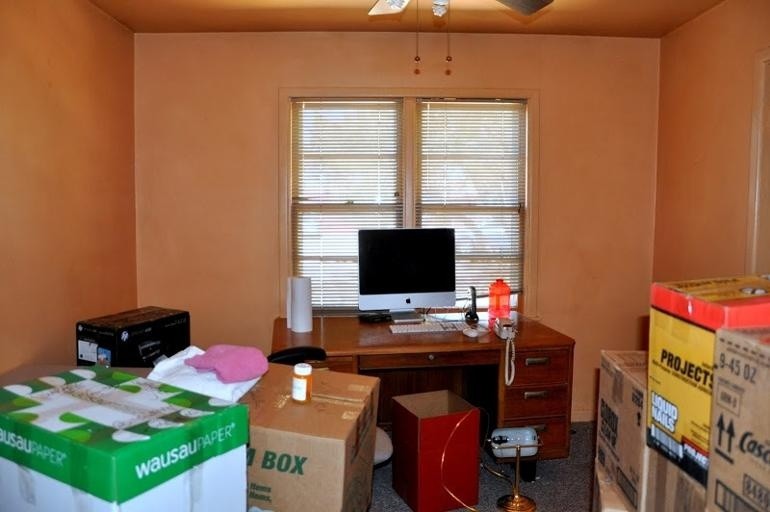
270 307 575 465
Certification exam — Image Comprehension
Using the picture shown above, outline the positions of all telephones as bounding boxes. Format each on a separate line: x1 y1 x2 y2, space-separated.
493 317 515 340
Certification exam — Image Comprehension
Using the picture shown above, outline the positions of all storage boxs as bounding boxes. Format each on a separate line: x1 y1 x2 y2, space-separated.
0 306 483 512
591 274 770 512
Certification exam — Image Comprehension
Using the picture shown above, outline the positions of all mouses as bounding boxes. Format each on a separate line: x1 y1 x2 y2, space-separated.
464 328 478 337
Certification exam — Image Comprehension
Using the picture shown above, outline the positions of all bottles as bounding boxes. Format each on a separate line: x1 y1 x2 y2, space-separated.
488 278 513 316
291 362 313 404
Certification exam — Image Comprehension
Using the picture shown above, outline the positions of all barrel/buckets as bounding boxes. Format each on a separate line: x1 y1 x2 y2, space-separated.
488 279 511 331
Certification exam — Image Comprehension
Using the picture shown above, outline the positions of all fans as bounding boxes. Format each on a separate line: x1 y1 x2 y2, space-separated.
368 0 554 63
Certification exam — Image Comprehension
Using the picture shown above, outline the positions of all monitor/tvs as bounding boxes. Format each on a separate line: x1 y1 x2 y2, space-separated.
357 228 456 323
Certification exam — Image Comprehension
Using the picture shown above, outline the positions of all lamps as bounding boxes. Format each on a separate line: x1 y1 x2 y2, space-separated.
488 422 544 512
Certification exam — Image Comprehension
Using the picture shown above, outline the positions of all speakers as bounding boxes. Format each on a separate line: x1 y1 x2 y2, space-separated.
467 286 477 315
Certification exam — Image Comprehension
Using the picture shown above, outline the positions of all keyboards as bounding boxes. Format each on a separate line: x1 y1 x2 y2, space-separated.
390 322 471 335
